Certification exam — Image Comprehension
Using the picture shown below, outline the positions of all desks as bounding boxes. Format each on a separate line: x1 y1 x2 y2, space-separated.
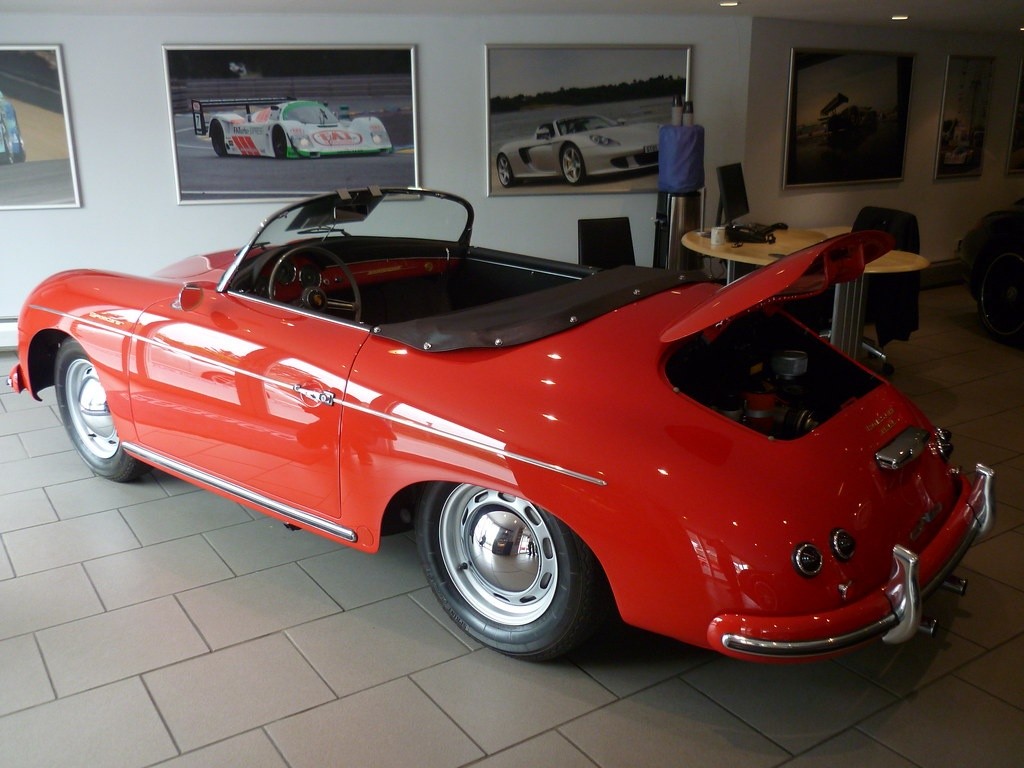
681 224 929 366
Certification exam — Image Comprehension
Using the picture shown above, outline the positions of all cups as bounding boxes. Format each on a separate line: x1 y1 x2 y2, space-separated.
683 101 694 127
671 95 683 127
740 384 776 434
711 227 725 245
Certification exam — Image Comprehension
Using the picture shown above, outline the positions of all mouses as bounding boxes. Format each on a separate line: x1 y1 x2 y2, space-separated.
770 222 788 230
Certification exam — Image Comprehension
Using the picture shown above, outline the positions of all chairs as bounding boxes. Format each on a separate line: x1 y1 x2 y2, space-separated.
816 207 920 375
578 218 635 271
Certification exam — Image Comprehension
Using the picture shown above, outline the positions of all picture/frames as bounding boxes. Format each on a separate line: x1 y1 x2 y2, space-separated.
934 54 995 179
1006 57 1024 175
488 44 694 197
162 44 420 206
782 48 917 190
0 45 83 211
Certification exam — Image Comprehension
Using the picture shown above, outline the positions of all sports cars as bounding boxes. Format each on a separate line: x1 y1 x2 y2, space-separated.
190 94 394 161
4 185 998 666
495 112 664 188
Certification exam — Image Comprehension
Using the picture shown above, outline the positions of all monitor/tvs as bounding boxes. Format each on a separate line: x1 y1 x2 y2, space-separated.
714 163 750 227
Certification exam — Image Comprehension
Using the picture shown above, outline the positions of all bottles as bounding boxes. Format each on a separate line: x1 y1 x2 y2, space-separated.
774 351 808 426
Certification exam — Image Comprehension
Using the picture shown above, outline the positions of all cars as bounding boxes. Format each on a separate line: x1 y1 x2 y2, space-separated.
960 195 1024 346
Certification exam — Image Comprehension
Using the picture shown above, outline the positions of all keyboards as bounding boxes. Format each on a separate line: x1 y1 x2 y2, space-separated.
748 223 773 235
